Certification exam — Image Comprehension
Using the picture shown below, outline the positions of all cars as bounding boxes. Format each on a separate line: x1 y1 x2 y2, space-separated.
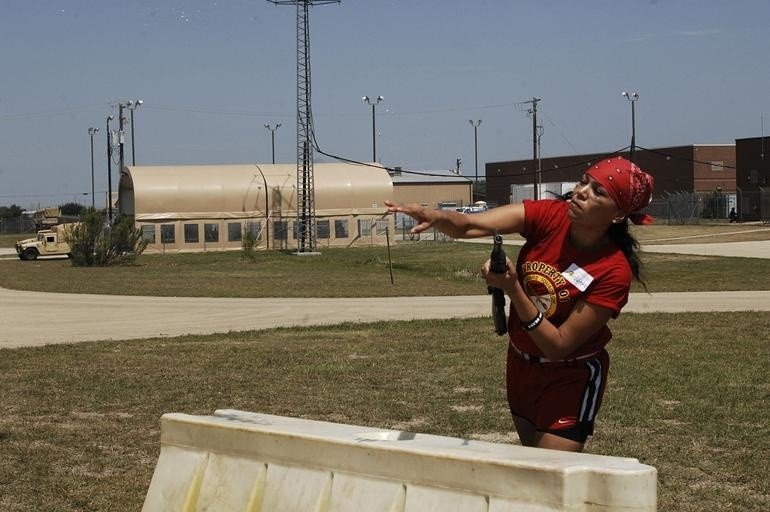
456 206 485 215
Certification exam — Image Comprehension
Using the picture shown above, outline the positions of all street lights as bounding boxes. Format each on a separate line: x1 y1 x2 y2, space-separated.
88 127 100 227
264 123 281 165
126 100 143 167
469 120 482 200
621 91 640 164
107 115 114 226
362 95 384 163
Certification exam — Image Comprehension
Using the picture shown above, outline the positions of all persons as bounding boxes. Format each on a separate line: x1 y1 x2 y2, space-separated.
384 156 656 453
729 207 737 223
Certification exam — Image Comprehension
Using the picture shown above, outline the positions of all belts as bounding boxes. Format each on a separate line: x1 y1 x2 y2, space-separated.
511 339 598 363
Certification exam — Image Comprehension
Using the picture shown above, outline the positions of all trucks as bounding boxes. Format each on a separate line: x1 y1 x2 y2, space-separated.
35 215 80 234
16 222 97 261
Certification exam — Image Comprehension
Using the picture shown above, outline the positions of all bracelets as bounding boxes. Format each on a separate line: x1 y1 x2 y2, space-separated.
521 312 543 331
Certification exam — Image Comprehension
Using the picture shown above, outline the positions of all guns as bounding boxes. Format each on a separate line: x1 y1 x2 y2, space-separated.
487 233 507 336
